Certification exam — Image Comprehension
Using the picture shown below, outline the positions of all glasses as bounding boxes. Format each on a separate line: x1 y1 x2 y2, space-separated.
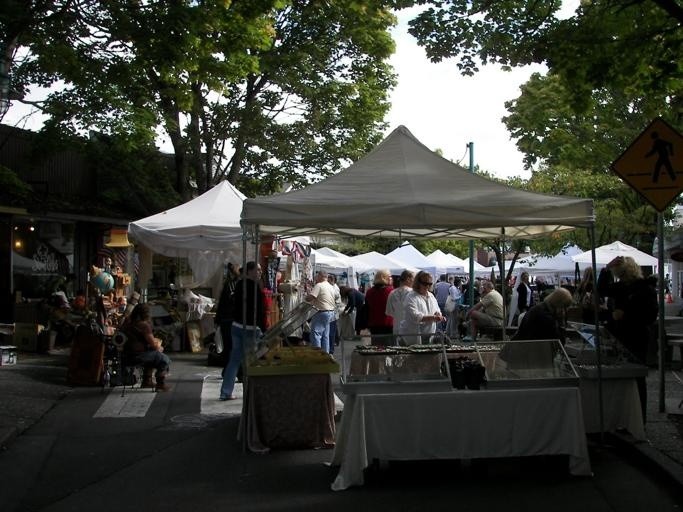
420 281 433 286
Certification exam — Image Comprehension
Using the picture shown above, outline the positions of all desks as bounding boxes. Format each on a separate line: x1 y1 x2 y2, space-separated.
238 344 339 450
341 389 585 484
576 376 638 439
345 342 498 382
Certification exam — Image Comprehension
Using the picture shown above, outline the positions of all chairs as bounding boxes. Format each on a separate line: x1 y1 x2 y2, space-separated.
102 333 156 395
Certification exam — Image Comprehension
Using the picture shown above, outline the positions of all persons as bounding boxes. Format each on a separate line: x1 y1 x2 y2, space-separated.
121 304 173 393
38 269 674 382
219 261 269 400
607 255 660 434
214 262 243 378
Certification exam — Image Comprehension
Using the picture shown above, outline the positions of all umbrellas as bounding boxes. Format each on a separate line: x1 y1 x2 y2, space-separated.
572 238 658 268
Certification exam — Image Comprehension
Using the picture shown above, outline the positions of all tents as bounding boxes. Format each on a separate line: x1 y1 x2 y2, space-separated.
293 239 589 289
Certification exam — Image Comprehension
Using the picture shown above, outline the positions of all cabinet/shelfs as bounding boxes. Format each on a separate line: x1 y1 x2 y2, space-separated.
337 329 581 390
564 318 649 380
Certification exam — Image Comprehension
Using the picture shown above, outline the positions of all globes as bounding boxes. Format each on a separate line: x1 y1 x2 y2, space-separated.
97 271 114 298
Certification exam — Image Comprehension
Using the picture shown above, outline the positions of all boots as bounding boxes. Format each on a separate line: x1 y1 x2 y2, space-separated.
140 374 156 388
155 376 173 392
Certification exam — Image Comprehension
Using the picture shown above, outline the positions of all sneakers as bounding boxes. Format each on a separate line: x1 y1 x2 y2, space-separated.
219 370 244 401
